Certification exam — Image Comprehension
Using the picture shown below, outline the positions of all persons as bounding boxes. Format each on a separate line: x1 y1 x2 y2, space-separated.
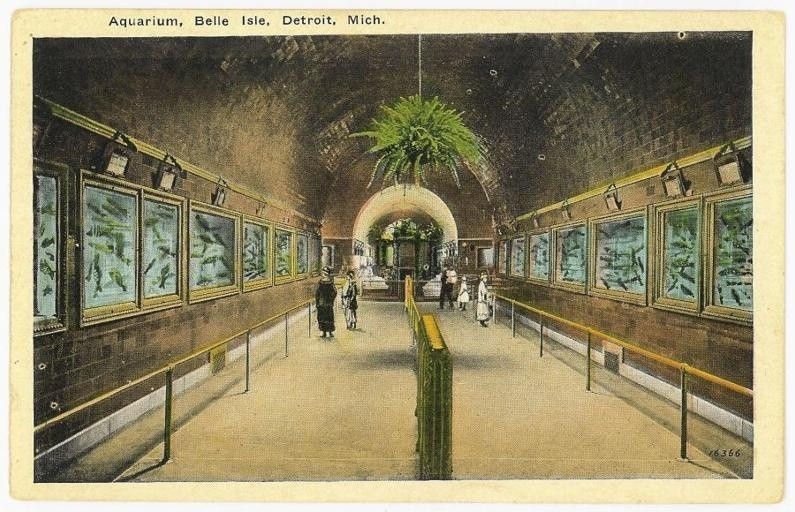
341 271 358 329
437 264 454 310
476 272 493 327
456 274 469 311
315 266 337 338
